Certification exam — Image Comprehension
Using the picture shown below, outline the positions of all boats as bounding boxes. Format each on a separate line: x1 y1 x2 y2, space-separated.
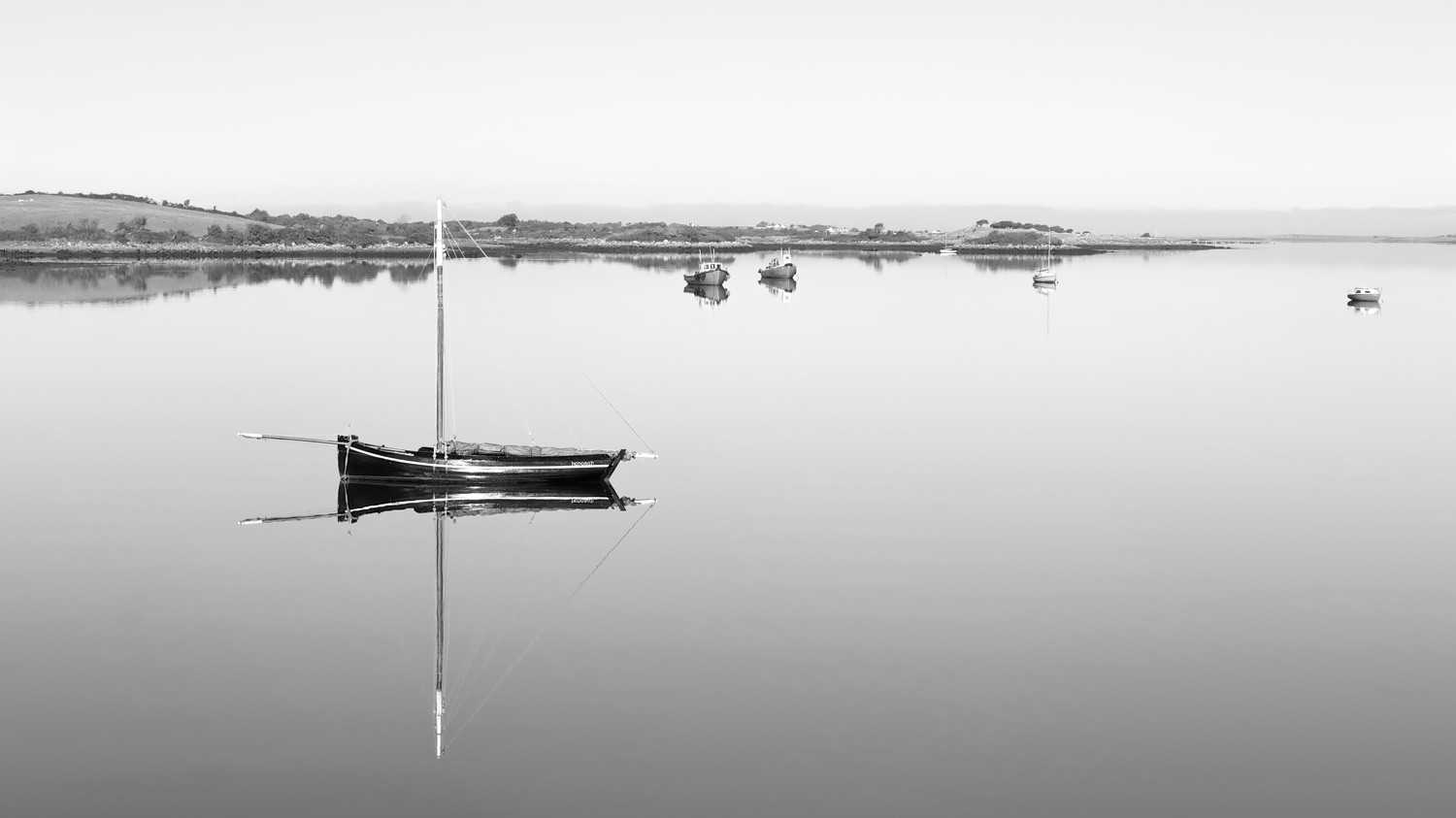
758 279 796 301
1347 287 1382 301
683 285 730 306
682 245 730 285
1347 302 1382 313
758 247 797 279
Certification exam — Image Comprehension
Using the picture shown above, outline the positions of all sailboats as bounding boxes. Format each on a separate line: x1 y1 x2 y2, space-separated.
1031 283 1056 328
1032 228 1057 282
237 197 660 482
238 482 658 759
